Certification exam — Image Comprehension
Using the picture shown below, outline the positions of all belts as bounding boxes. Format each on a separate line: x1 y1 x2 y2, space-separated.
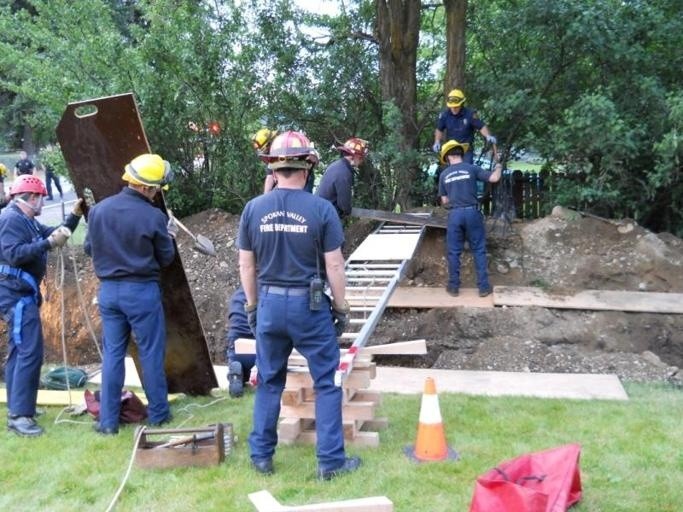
452 205 475 209
261 285 309 296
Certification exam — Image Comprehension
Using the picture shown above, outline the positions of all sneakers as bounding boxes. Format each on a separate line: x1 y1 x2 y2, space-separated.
7 419 44 438
7 408 44 417
94 423 118 433
479 285 492 297
447 286 458 296
319 458 361 480
255 459 272 473
149 414 173 427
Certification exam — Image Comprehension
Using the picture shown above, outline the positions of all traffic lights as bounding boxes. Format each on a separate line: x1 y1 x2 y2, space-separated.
210 122 220 136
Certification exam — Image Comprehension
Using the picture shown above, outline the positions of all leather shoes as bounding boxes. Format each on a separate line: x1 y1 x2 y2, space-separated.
229 361 243 398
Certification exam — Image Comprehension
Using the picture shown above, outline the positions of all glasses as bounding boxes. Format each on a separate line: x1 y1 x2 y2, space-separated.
163 160 175 184
447 96 460 103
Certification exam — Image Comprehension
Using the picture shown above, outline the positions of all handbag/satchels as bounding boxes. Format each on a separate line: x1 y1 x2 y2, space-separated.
85 390 148 422
44 367 87 390
468 444 584 512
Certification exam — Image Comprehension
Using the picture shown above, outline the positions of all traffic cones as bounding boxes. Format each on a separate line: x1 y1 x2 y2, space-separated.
405 376 458 463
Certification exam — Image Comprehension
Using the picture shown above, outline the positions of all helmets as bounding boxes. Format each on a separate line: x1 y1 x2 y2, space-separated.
446 90 466 107
10 175 47 195
337 138 371 156
122 154 176 191
252 129 279 150
258 131 319 170
0 165 10 178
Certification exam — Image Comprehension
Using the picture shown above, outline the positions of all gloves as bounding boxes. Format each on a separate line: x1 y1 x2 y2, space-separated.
432 141 442 152
47 225 71 248
330 300 349 337
71 198 83 216
244 302 256 338
487 136 497 143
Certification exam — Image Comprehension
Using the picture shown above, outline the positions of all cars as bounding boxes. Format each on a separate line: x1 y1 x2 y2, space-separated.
420 157 512 196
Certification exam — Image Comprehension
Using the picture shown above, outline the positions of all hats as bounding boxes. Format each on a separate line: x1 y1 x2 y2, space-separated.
440 139 470 165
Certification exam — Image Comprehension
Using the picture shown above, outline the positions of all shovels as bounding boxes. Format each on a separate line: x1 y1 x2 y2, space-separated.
165 208 217 257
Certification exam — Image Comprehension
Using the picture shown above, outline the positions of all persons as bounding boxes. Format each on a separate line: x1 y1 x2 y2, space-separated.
233 128 366 483
313 136 369 254
251 127 314 197
0 147 61 206
435 139 504 298
433 87 498 168
0 174 83 435
225 280 258 400
85 153 180 436
